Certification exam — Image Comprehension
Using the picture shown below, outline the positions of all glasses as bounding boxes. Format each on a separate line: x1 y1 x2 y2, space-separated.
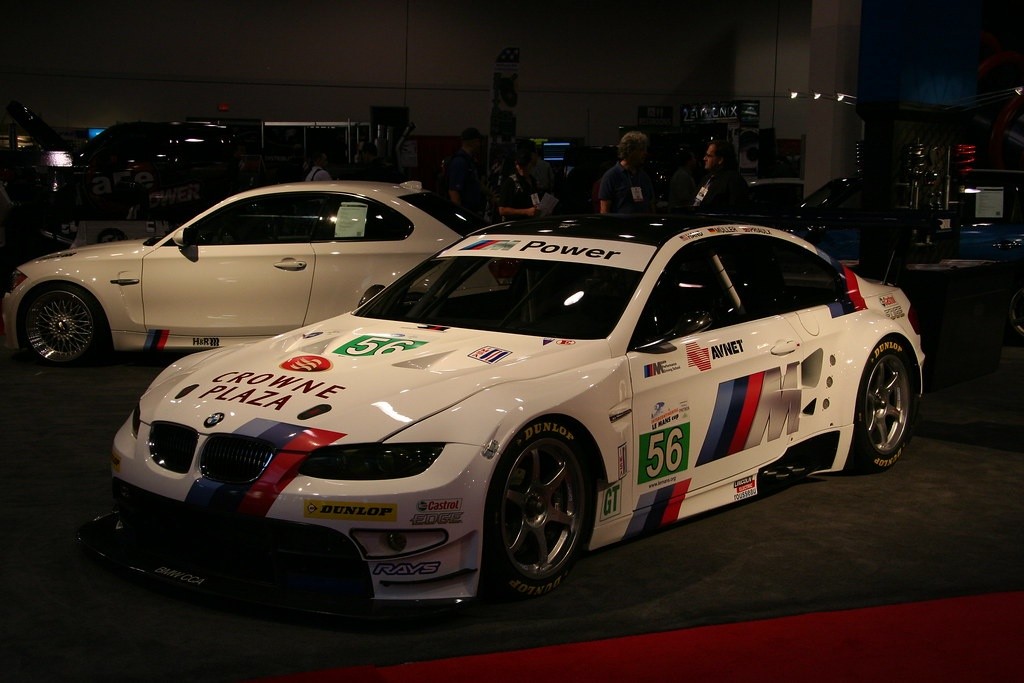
705 153 719 157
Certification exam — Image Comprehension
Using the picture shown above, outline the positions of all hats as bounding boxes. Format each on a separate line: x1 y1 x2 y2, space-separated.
462 128 483 141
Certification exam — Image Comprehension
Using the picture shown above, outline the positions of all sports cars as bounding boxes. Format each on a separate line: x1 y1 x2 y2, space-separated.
0 178 485 369
107 215 933 603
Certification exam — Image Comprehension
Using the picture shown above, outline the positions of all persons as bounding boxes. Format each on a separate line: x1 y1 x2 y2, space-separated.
340 141 406 185
304 148 333 183
596 130 658 215
689 137 753 216
424 118 565 237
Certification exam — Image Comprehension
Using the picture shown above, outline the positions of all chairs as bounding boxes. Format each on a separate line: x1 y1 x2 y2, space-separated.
504 260 787 358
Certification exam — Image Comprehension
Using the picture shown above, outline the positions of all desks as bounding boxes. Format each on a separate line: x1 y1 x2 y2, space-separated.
905 259 1007 390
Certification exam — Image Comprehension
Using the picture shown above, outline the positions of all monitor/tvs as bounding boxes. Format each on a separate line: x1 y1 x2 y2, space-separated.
542 140 571 160
974 184 1005 220
88 128 106 140
